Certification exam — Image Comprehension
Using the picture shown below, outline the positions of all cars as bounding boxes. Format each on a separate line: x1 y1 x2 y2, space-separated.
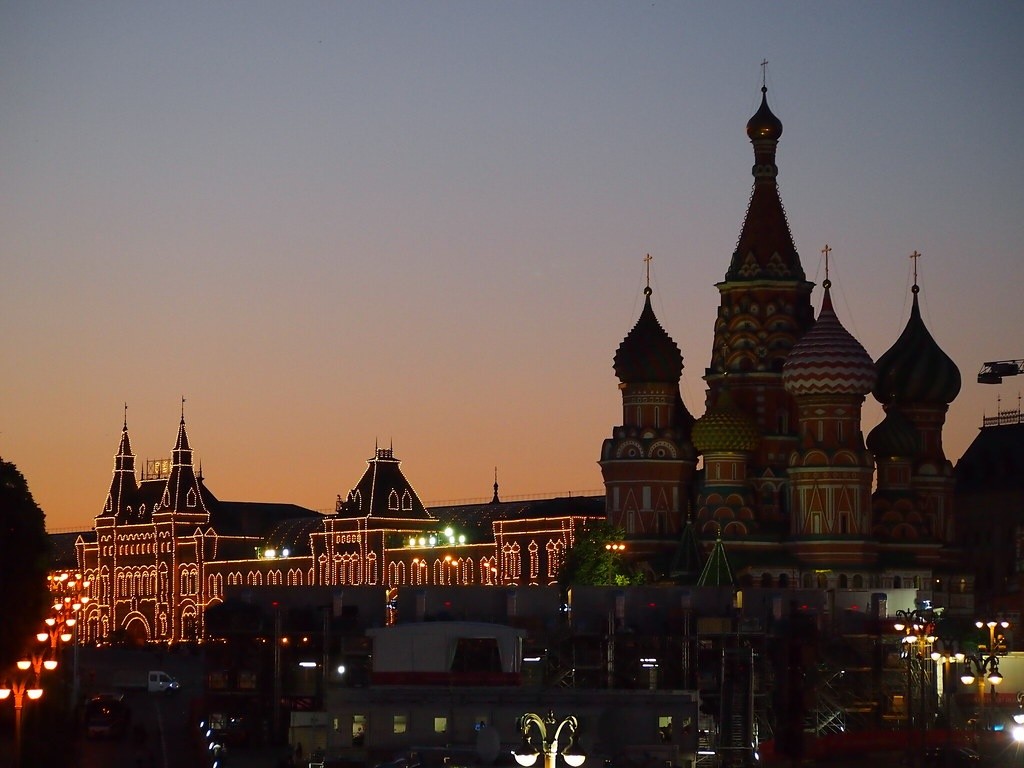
85 694 129 736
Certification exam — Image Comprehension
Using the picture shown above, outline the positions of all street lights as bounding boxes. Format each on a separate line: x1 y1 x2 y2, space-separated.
960 655 1003 712
0 671 43 768
894 610 935 755
17 618 76 707
976 619 1009 708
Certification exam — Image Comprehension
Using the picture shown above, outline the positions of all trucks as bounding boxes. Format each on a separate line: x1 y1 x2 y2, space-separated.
112 669 184 697
